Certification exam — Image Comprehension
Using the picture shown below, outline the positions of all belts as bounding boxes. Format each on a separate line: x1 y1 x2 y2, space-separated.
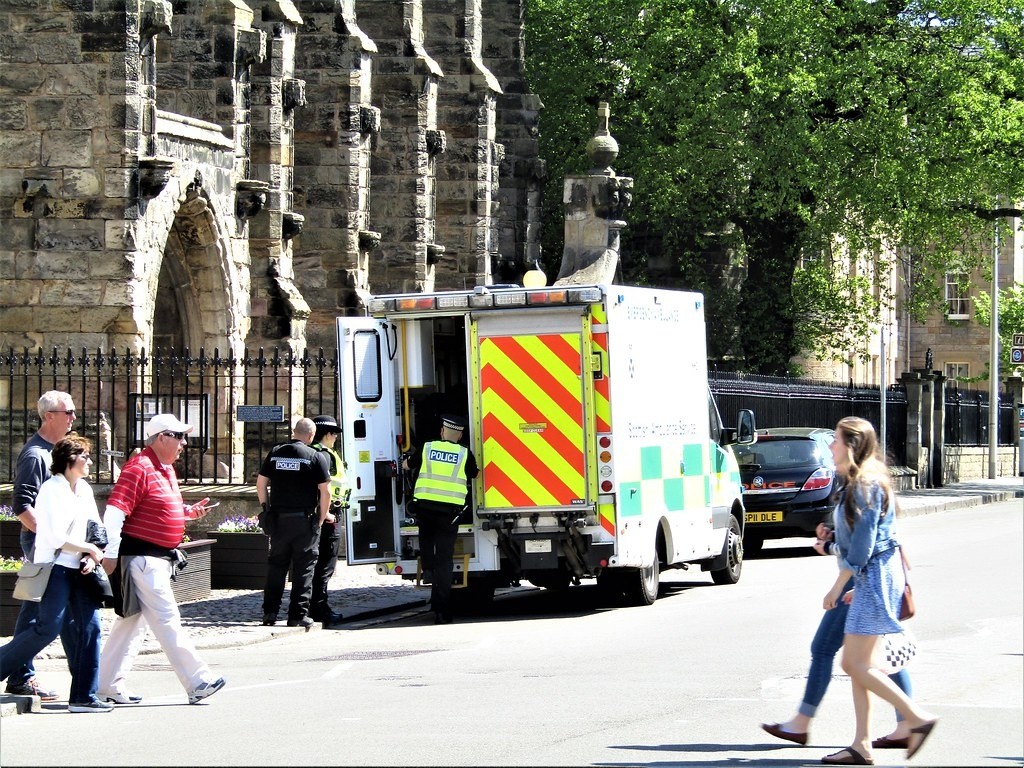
279 512 307 518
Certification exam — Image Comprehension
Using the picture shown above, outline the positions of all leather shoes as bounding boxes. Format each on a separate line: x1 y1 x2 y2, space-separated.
315 614 344 622
433 618 452 625
288 618 310 627
263 614 277 627
423 571 433 586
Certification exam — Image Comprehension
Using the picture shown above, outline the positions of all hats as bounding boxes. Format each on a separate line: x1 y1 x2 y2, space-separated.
313 415 342 433
145 413 193 440
442 414 467 431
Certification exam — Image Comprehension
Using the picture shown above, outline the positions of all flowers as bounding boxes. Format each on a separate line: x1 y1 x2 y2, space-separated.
0 555 24 571
183 531 194 542
0 505 20 520
215 515 258 532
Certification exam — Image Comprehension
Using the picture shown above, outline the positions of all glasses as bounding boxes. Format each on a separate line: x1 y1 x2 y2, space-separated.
47 409 78 415
75 454 90 460
162 432 189 440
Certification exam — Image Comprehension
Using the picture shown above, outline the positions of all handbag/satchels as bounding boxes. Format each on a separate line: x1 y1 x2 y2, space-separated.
898 584 915 622
12 561 54 602
870 629 917 674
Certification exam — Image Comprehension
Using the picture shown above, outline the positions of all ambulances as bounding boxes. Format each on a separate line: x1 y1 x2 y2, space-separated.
335 281 758 616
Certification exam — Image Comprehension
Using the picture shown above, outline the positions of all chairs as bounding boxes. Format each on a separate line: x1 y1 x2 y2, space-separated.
788 443 810 462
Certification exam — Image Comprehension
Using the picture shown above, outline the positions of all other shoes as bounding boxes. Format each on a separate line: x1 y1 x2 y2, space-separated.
762 724 809 745
873 736 910 749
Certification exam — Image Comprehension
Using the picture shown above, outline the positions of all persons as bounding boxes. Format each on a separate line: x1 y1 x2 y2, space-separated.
313 415 350 619
257 418 332 625
0 435 115 712
6 391 101 698
760 417 939 766
404 416 480 623
96 413 226 703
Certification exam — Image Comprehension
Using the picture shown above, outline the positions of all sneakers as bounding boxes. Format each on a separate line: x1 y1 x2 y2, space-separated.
189 676 225 706
66 698 116 712
6 681 60 700
99 693 141 704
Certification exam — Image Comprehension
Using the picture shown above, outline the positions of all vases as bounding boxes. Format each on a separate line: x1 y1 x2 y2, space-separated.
170 538 217 604
207 530 270 590
0 572 22 637
0 521 24 559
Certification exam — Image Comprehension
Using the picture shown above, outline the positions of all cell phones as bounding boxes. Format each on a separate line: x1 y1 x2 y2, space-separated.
203 502 220 509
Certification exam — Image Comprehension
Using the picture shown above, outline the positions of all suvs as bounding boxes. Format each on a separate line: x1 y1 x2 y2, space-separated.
731 427 847 560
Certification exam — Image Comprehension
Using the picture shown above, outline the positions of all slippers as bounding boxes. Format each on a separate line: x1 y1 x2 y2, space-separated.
906 719 942 760
822 747 874 765
